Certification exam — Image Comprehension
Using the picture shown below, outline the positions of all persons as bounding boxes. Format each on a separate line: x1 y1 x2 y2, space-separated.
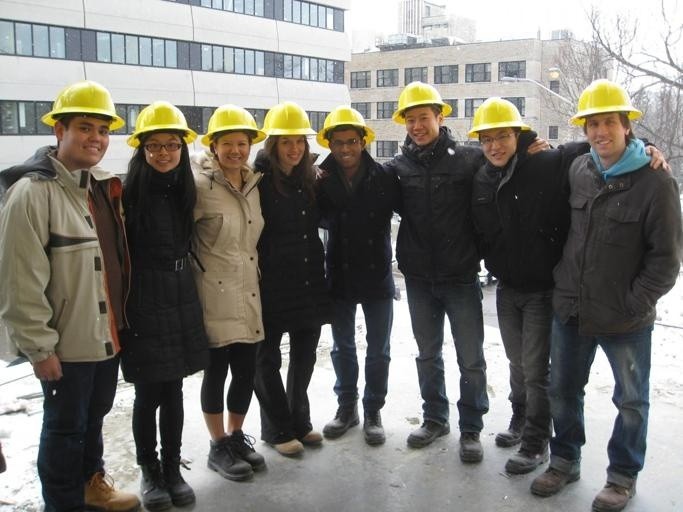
251 103 327 456
315 107 399 445
0 81 141 510
189 105 268 481
467 97 671 474
529 79 682 511
120 101 210 510
314 81 549 464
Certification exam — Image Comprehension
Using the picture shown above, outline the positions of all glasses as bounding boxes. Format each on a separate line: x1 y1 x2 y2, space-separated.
144 143 183 153
480 132 516 144
331 138 361 146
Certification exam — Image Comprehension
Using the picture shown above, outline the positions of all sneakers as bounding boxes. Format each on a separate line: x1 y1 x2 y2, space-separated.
460 432 483 462
275 439 304 456
505 445 549 474
495 429 521 448
592 481 636 512
531 466 580 497
301 431 323 445
407 420 450 448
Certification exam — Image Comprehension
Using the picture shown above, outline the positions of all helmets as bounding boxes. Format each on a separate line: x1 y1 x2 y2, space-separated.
41 80 125 131
262 103 318 136
200 104 268 146
392 81 452 125
467 97 531 138
316 108 375 148
570 80 643 126
127 101 198 148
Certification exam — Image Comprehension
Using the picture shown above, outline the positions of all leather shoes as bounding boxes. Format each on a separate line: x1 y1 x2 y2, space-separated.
324 399 360 438
364 408 386 446
208 437 254 481
225 429 266 471
140 460 173 512
84 472 141 511
162 459 196 508
